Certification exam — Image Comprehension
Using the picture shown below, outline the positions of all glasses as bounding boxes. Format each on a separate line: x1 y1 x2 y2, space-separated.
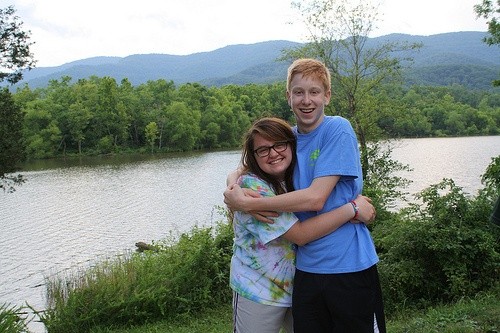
252 141 290 158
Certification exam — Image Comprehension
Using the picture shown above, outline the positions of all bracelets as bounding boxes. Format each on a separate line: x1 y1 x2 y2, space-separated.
350 200 359 220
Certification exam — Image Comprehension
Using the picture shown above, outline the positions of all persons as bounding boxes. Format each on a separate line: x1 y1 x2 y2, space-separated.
227 58 386 333
229 117 377 333
